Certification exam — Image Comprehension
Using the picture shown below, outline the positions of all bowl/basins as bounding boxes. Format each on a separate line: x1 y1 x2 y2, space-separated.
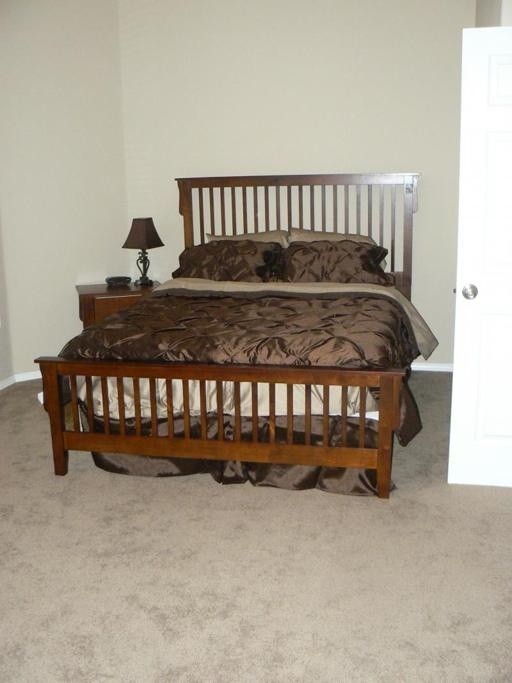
106 277 131 288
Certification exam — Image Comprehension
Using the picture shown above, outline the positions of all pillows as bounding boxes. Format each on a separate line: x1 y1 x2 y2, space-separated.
171 226 396 287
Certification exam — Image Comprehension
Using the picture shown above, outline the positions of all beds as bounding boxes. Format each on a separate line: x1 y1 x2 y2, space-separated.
34 172 423 498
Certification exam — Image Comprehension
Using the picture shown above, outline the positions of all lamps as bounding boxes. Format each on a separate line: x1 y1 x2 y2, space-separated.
122 217 165 287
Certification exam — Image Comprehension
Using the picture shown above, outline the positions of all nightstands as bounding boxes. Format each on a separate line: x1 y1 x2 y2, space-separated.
75 280 162 329
